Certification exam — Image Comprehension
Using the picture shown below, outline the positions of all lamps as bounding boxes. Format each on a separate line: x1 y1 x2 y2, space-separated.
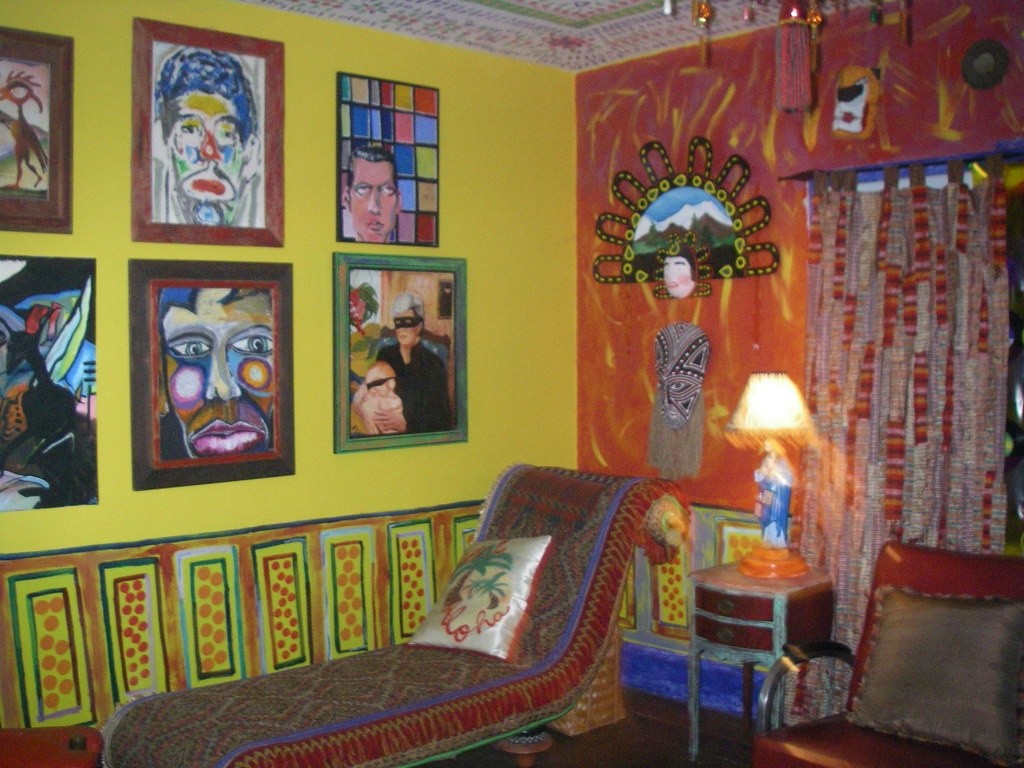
726 372 815 580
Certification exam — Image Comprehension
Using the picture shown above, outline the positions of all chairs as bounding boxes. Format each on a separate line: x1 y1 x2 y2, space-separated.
101 460 688 767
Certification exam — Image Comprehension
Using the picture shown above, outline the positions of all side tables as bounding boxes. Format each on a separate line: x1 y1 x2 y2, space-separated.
689 561 833 763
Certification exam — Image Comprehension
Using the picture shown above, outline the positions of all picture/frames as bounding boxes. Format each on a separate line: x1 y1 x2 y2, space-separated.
131 16 286 247
331 251 469 455
127 257 297 492
0 25 75 236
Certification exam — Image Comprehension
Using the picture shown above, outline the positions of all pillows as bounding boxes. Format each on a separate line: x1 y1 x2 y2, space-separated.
401 534 554 665
844 582 1024 768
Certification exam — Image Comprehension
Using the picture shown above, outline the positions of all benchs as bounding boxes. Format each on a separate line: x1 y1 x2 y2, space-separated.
748 541 1024 768
